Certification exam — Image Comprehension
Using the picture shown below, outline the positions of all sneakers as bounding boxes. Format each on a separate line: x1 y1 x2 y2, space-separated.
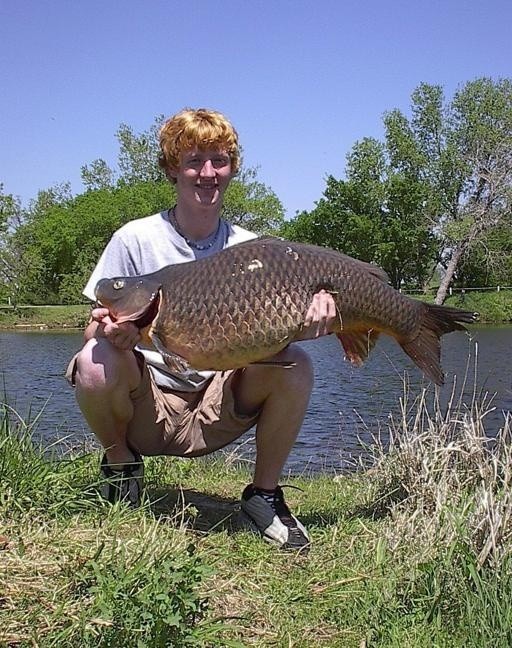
99 448 144 508
241 483 312 554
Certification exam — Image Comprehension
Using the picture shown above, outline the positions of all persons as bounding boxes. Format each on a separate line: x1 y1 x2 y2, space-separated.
65 108 337 552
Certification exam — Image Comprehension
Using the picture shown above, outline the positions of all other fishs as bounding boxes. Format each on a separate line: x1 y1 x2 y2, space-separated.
94 235 481 387
